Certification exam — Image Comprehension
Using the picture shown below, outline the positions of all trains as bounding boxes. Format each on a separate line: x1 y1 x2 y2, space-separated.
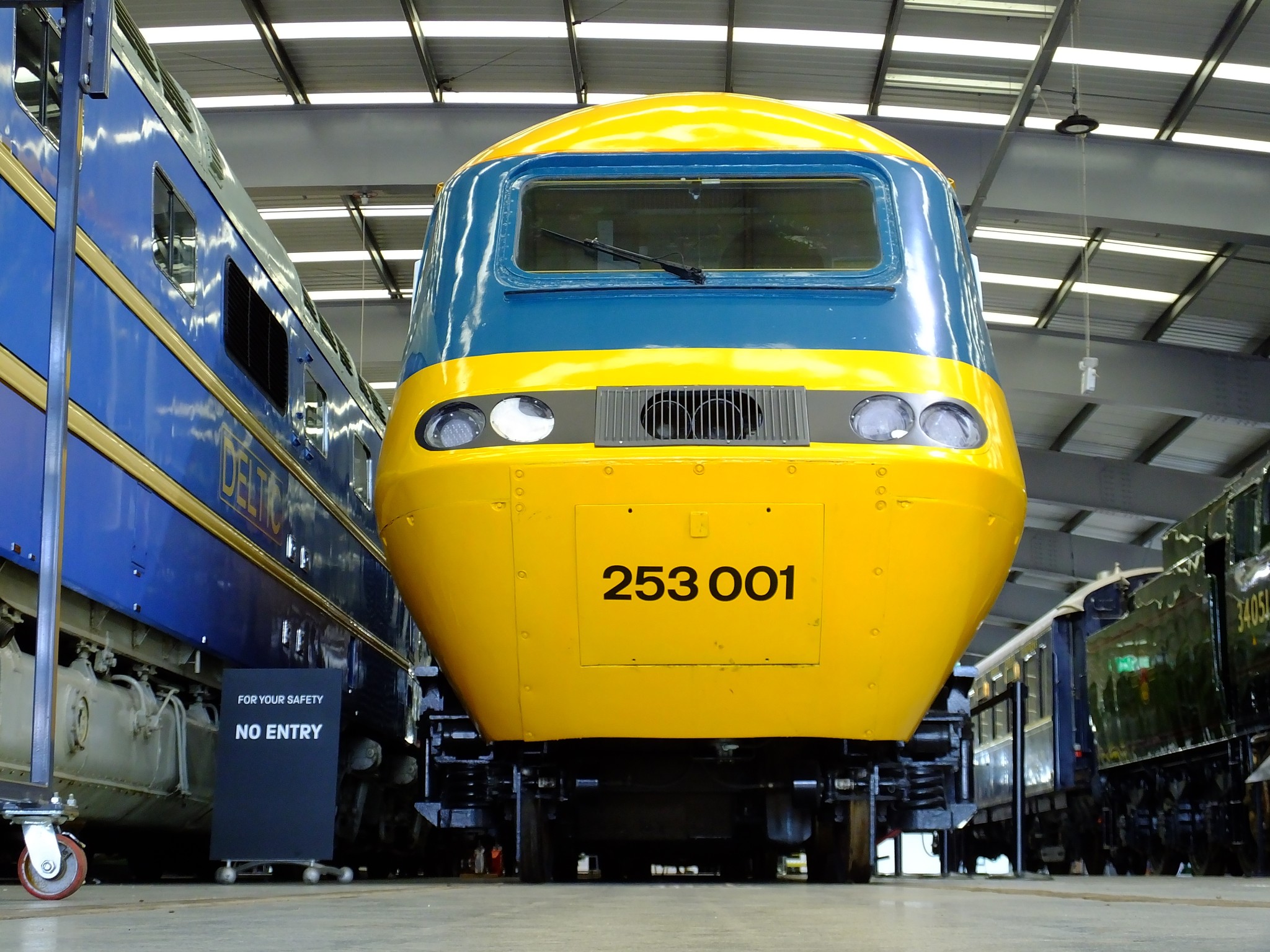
376 92 1028 884
0 0 447 901
932 451 1270 879
